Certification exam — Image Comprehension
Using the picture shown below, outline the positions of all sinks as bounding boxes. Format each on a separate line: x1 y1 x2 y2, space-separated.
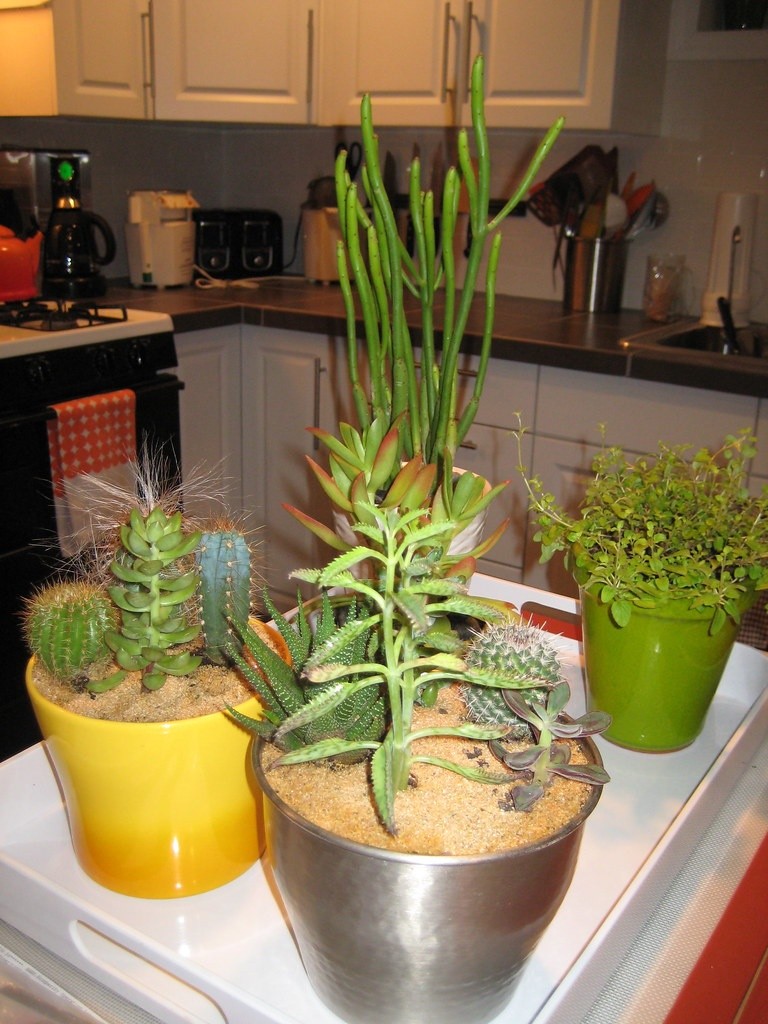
618 320 768 364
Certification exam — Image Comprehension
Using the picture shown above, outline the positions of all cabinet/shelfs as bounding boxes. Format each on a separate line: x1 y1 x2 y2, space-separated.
0 0 620 140
158 323 768 651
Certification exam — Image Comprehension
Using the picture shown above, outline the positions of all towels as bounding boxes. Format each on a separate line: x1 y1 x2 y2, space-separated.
45 389 140 559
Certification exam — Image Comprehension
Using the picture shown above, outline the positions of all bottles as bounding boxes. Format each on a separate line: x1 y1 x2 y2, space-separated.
641 252 685 324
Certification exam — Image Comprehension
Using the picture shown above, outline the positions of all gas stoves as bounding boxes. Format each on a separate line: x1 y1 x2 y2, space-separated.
0 302 179 413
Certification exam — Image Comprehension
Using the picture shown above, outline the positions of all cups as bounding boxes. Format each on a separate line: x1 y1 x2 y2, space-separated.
563 237 628 314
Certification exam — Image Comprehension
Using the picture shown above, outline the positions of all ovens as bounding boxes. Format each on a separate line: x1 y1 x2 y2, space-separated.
0 372 187 763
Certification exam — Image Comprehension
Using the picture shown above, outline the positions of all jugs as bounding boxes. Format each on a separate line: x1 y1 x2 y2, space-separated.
42 196 117 301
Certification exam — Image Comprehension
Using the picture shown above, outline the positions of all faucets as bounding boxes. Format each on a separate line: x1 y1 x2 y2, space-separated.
728 224 742 307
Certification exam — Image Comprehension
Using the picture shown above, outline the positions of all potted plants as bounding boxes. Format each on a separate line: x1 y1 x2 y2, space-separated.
220 410 614 1024
19 432 294 900
507 409 768 754
333 57 564 591
